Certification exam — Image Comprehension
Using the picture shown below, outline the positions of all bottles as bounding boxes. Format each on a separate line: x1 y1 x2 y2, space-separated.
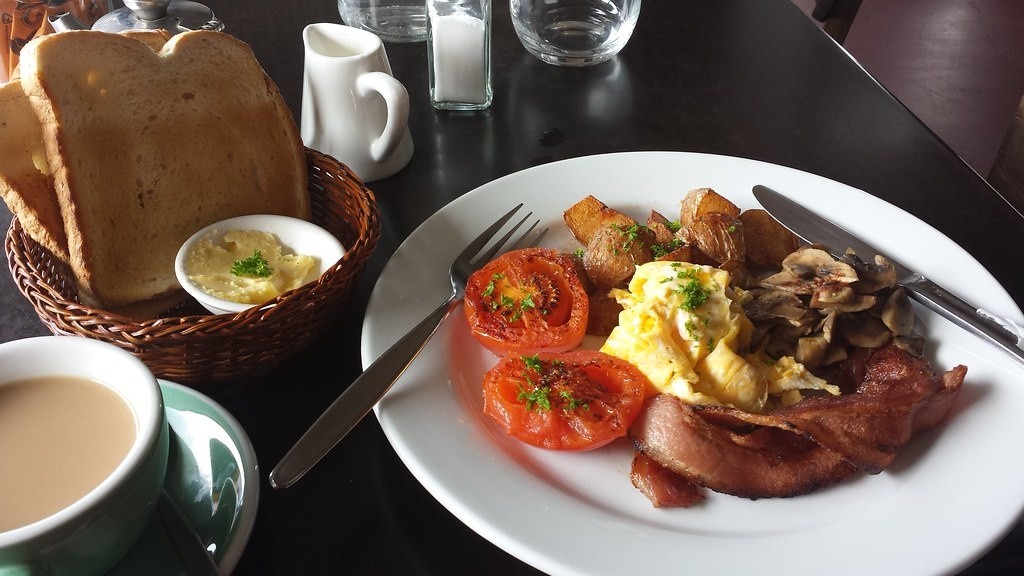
425 0 492 110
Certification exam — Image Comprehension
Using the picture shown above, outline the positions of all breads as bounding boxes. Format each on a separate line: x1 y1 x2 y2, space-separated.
0 27 307 307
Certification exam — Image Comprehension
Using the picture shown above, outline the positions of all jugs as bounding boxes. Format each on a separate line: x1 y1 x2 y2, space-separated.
46 1 225 42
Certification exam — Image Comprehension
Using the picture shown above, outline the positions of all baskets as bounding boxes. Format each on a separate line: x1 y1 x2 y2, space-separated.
3 145 384 385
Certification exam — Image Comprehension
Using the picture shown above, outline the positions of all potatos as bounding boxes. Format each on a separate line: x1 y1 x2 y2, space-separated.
563 186 800 337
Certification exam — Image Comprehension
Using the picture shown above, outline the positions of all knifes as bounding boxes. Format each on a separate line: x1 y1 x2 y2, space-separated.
752 185 1024 366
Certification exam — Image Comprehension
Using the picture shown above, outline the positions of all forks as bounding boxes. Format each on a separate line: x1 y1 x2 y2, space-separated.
269 202 548 490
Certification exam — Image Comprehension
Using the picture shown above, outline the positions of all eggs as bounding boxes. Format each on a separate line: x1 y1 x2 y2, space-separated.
599 259 842 416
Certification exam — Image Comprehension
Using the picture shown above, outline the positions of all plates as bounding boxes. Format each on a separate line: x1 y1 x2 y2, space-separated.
173 214 346 318
361 152 1024 574
101 376 260 575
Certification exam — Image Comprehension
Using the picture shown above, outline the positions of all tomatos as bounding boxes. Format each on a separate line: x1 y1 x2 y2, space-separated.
460 246 646 452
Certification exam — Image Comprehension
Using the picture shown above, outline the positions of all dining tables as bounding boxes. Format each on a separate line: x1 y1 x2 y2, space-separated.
0 0 1024 576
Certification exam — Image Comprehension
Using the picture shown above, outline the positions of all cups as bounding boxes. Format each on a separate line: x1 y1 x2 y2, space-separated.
298 23 416 184
509 1 642 68
1 337 169 576
336 0 430 45
